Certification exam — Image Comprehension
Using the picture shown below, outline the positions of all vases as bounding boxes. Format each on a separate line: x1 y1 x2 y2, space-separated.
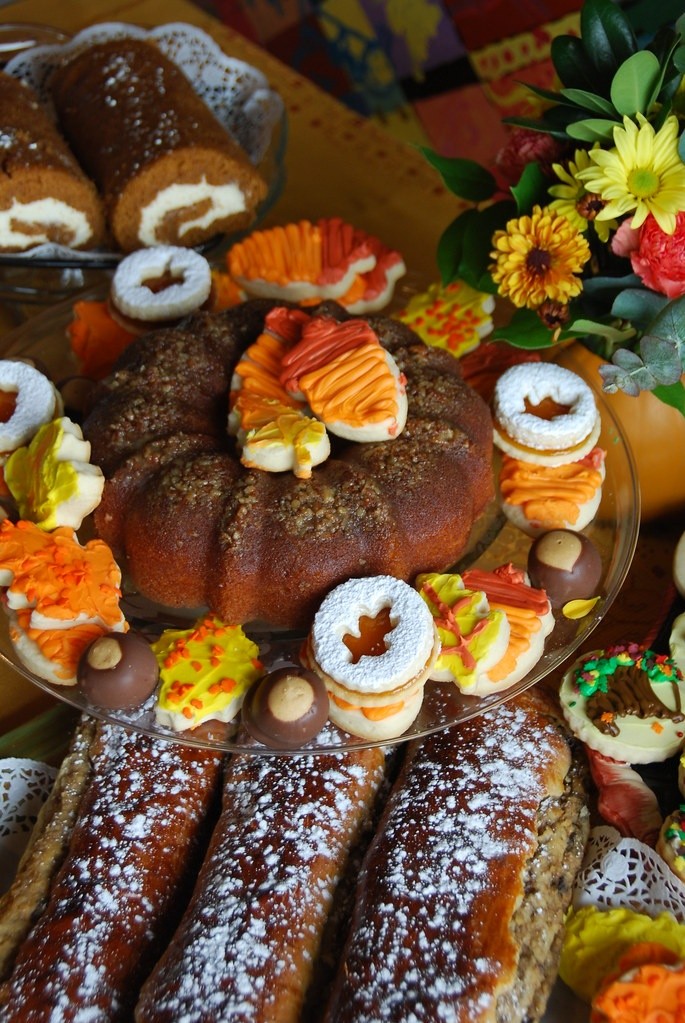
553 346 685 525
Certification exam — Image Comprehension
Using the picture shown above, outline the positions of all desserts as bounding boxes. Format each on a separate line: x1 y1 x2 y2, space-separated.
0 221 685 1023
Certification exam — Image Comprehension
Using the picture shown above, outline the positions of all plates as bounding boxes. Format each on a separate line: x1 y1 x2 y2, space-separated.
0 24 291 271
0 290 641 753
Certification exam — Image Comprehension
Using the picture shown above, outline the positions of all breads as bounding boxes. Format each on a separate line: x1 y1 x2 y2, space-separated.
0 27 592 1023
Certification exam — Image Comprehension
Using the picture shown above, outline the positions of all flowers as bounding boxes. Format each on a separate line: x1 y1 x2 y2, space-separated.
406 0 685 416
